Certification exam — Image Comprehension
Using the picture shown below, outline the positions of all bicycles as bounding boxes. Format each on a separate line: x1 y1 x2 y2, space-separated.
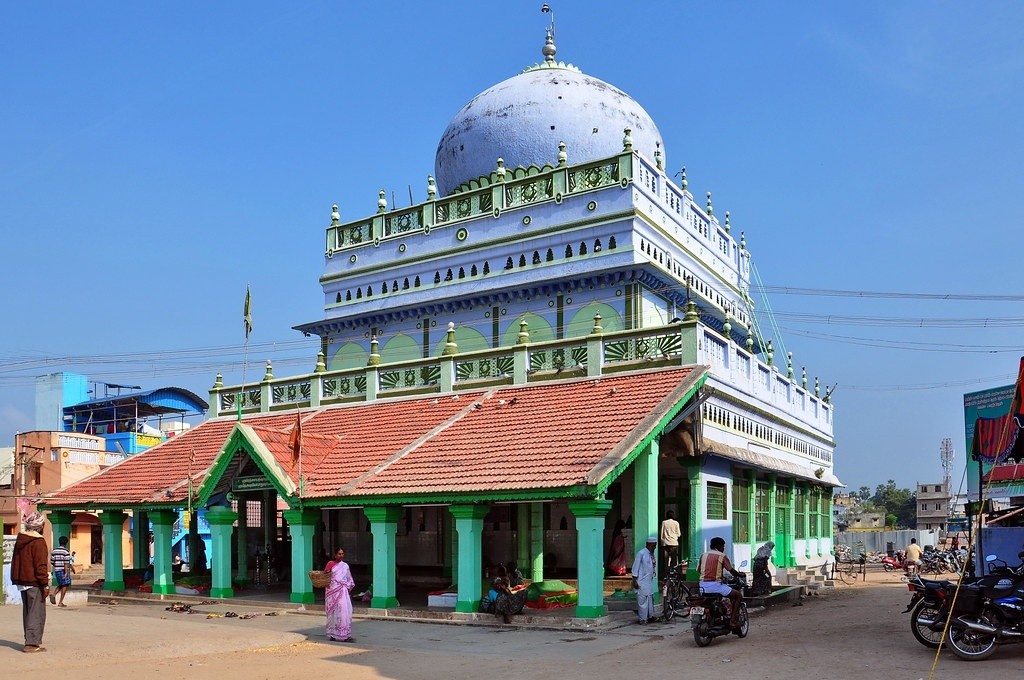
662 559 693 624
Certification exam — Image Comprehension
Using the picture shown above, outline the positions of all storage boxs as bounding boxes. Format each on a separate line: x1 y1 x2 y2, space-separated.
948 586 980 611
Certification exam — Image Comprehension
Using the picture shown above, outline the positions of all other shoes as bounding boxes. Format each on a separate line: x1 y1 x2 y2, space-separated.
330 636 335 641
729 621 744 627
648 616 657 623
640 620 647 625
345 637 354 642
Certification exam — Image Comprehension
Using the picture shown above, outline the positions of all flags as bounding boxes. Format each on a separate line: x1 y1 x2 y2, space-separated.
243 290 253 336
288 410 302 467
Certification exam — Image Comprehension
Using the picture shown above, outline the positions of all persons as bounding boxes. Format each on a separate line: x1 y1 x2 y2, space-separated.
492 561 528 623
903 537 923 578
11 513 48 652
606 510 681 626
951 537 976 568
750 542 776 595
144 529 207 580
316 548 357 643
697 538 746 627
50 536 77 608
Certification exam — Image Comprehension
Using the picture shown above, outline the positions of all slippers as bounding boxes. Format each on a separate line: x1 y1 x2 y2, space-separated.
58 603 67 607
50 593 56 604
23 646 46 653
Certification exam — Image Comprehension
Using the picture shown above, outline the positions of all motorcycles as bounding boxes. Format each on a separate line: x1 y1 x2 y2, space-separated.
903 554 924 582
902 554 1018 650
685 559 749 647
866 531 975 575
923 560 1024 661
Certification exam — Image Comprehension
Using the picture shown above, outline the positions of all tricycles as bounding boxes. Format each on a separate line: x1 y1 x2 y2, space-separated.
834 540 867 585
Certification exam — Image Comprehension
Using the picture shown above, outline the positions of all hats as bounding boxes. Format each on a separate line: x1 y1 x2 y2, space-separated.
646 537 657 542
25 512 45 529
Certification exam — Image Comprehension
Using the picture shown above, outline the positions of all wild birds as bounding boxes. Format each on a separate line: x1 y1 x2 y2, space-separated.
552 365 564 379
609 387 618 396
526 368 539 375
669 317 681 324
428 371 517 410
577 362 585 370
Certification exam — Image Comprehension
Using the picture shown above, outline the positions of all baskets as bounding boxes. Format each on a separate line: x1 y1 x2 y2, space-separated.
308 571 333 589
